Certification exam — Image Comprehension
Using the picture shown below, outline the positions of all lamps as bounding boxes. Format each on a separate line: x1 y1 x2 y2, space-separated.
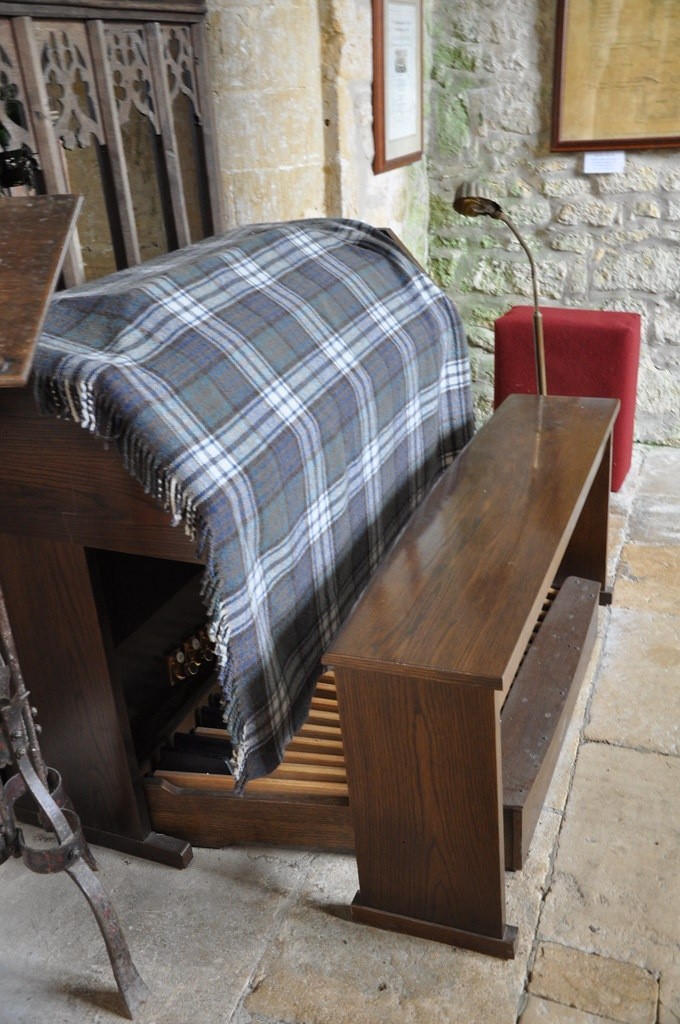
453 180 503 222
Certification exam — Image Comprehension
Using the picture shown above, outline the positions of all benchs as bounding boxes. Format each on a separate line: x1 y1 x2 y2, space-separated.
321 394 622 962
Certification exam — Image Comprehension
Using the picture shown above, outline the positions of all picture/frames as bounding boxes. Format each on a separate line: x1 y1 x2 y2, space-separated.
549 1 680 153
371 0 424 175
